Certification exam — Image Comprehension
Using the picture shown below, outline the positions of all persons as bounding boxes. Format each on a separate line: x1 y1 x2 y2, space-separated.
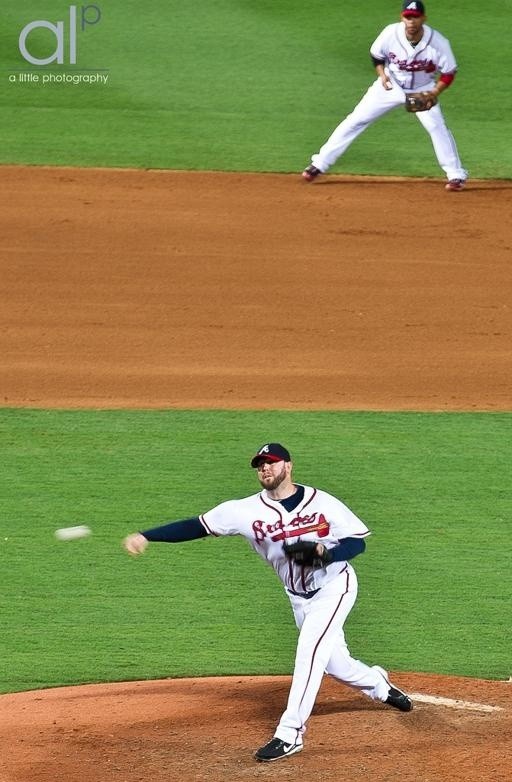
300 0 470 192
122 443 414 761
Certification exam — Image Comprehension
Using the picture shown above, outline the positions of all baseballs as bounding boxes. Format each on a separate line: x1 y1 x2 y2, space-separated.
55 525 91 543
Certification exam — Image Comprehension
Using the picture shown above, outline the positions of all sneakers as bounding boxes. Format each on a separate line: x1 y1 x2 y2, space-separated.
446 178 467 191
302 164 321 182
372 665 413 712
254 736 303 762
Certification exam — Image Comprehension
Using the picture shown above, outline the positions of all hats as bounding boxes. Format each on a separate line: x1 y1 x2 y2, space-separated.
252 443 291 468
401 1 425 18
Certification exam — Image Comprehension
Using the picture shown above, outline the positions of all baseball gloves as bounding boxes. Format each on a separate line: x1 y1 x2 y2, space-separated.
404 93 437 113
283 541 333 567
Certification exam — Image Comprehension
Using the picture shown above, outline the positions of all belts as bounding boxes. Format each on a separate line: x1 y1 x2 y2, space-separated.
288 587 320 599
